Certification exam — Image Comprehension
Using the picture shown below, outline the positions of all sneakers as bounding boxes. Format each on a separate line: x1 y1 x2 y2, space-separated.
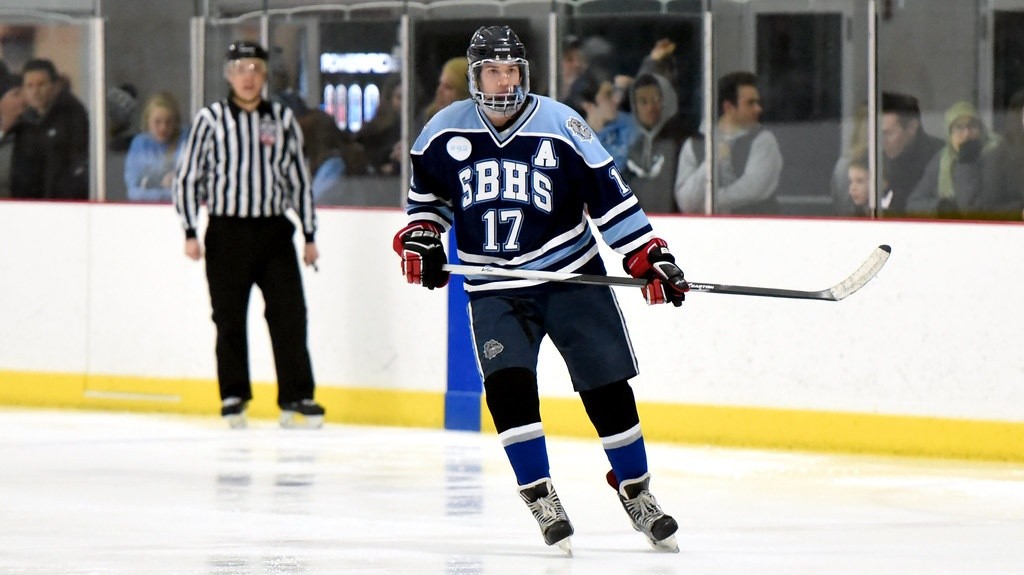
517 477 574 558
278 399 323 429
220 396 249 428
607 469 680 554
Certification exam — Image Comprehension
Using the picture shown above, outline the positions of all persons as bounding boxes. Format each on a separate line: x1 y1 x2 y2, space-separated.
824 93 1024 221
0 59 89 201
123 93 186 201
560 32 689 215
172 40 326 429
392 24 689 558
413 57 470 136
674 71 785 214
108 83 142 151
285 71 423 206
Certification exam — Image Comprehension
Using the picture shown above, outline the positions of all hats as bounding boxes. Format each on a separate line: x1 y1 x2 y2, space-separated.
0 61 24 100
225 40 267 61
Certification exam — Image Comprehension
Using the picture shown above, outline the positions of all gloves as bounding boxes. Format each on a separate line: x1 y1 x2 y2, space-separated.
621 238 688 307
393 223 449 290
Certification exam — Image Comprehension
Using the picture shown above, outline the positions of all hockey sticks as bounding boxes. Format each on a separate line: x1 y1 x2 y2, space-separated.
444 244 892 302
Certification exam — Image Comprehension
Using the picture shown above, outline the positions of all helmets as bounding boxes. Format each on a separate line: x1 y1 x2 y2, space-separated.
466 25 530 116
